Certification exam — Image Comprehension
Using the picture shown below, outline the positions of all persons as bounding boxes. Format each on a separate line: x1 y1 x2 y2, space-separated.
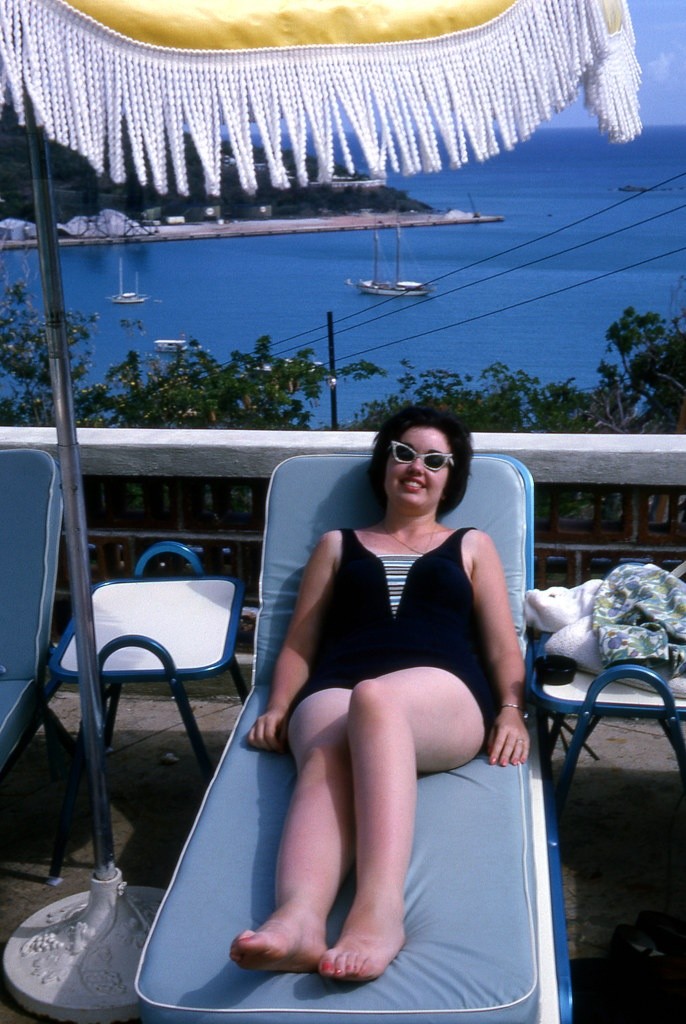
231 406 530 978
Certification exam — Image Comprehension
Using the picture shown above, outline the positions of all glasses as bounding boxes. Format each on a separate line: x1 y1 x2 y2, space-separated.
387 441 454 471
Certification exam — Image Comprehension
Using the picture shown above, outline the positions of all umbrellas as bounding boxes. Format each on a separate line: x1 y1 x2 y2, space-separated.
0 1 644 1006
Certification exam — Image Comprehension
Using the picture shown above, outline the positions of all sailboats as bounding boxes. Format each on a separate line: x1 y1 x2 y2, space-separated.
345 197 439 297
106 257 145 303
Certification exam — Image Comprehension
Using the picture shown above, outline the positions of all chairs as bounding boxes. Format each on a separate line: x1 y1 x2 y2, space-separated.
131 454 573 1024
0 448 63 773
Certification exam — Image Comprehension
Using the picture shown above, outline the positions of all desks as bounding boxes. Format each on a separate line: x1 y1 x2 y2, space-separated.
529 634 686 825
45 541 249 887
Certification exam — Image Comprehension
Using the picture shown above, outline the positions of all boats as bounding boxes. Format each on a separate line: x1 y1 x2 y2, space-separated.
247 358 324 380
153 339 188 351
617 185 645 191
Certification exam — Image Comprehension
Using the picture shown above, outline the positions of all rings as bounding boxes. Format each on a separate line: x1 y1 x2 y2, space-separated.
517 739 524 742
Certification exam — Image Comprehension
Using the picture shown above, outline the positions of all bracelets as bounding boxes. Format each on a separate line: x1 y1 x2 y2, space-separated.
501 704 520 708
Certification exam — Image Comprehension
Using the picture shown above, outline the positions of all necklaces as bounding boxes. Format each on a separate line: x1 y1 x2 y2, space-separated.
382 522 435 554
